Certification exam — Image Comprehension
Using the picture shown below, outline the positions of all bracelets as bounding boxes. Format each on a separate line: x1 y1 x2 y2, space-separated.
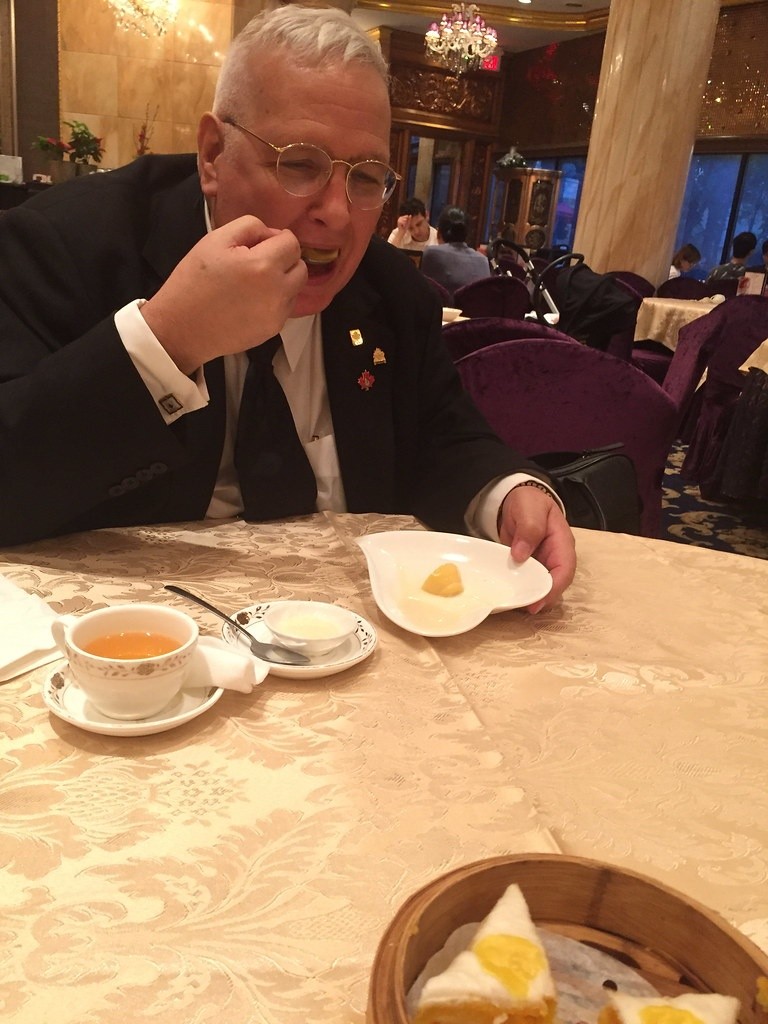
497 482 553 521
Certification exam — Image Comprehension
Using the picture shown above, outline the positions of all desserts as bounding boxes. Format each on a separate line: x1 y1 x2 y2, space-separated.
596 989 742 1024
414 882 558 1024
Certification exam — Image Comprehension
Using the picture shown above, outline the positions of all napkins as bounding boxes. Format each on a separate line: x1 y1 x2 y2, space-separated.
182 633 273 693
0 572 66 684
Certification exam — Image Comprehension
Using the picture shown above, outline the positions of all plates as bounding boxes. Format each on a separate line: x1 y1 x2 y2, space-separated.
354 530 553 637
222 600 378 680
41 635 225 737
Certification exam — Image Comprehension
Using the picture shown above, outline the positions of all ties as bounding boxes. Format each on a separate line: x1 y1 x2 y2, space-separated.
231 333 320 522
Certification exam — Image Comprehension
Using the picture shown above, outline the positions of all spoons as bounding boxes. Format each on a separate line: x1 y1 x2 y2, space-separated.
300 256 333 264
164 585 311 665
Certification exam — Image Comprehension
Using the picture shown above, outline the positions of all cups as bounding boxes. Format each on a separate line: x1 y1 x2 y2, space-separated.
65 604 200 720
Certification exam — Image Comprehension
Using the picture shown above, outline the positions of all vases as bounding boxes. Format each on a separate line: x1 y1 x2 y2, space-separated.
48 160 98 188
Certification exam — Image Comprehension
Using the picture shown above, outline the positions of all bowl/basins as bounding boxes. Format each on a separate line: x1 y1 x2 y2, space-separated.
264 602 358 656
442 307 462 321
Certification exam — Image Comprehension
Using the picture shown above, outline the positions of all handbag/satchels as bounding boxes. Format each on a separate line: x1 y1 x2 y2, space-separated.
527 442 644 537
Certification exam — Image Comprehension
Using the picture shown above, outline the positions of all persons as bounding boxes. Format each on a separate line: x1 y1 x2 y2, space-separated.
0 3 577 616
756 240 768 289
706 232 757 280
419 206 491 295
668 244 701 281
387 198 439 251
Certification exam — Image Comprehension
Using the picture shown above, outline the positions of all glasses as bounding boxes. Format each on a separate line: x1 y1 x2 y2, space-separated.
223 117 402 211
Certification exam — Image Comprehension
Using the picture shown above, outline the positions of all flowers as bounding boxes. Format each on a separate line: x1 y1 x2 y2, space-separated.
26 117 108 165
134 100 156 156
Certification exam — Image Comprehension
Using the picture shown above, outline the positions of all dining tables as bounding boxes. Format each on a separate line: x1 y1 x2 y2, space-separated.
0 512 768 1024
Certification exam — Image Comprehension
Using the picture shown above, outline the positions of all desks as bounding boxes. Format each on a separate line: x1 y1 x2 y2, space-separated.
635 297 725 353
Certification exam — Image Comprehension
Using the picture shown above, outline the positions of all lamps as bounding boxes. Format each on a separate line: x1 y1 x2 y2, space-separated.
423 1 499 79
104 0 183 40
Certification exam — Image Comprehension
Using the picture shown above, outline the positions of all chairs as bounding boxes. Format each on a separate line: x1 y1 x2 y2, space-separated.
395 244 768 540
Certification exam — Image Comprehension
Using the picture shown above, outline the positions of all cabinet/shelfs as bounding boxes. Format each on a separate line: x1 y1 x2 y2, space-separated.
488 166 563 251
